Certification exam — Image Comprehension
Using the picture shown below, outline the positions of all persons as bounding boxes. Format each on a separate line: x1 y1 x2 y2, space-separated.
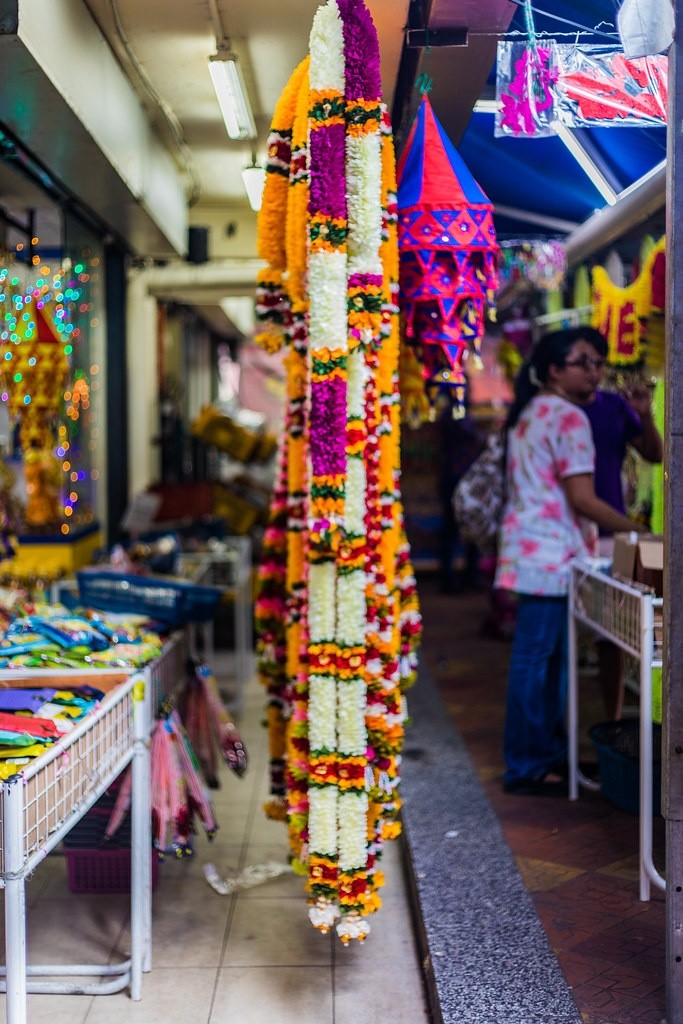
493 323 665 797
437 393 490 593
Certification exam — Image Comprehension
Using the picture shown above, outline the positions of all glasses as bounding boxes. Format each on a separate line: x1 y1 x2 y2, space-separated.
566 351 604 372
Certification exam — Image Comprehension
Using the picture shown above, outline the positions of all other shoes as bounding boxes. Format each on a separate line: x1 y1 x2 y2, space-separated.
503 769 581 797
550 752 601 779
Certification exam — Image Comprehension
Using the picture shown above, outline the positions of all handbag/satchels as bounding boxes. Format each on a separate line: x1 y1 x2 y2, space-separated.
452 433 506 538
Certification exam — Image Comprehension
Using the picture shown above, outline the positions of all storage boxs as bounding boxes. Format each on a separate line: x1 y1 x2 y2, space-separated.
613 533 663 588
63 772 159 897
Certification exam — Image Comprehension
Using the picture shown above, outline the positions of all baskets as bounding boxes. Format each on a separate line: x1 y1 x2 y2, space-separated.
76 569 226 627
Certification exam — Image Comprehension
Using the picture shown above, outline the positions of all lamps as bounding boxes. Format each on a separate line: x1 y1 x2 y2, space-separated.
208 39 257 140
243 155 268 212
